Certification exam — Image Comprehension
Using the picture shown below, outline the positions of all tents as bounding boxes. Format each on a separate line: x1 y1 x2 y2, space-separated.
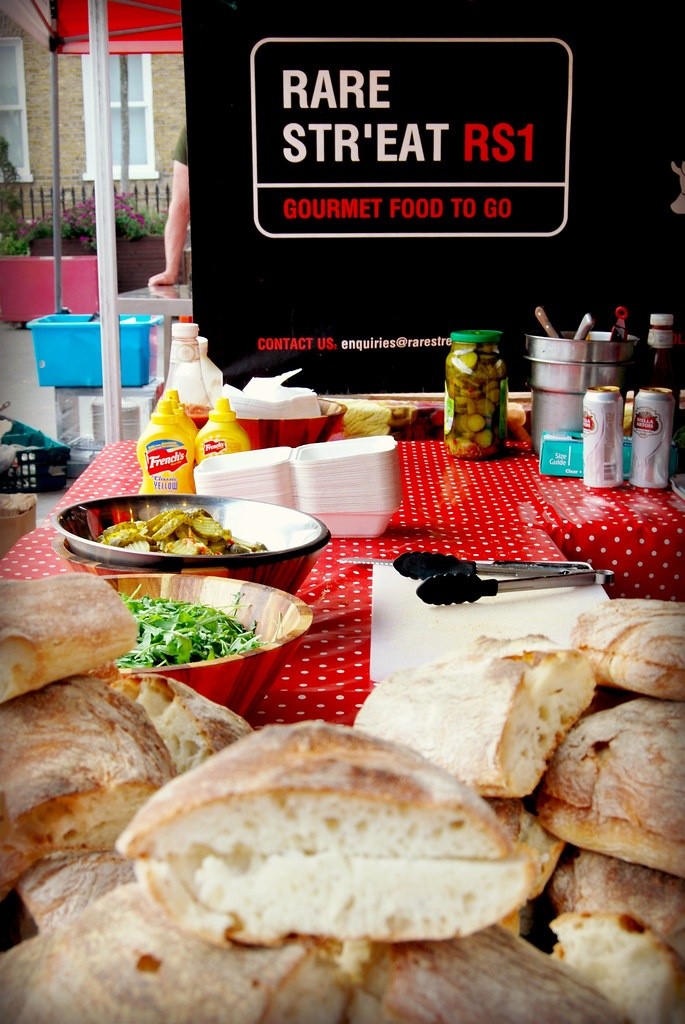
0 0 182 316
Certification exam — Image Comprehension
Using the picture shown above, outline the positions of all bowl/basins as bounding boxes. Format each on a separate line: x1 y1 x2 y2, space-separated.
52 533 332 596
97 573 313 724
51 492 329 575
193 434 402 539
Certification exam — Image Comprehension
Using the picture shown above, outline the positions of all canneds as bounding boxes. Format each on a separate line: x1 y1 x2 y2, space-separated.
630 387 676 489
444 331 509 460
581 385 624 488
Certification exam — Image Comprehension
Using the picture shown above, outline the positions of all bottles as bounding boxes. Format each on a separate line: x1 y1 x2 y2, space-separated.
196 337 223 410
194 397 251 466
634 312 681 437
161 315 213 408
167 389 200 463
136 400 196 495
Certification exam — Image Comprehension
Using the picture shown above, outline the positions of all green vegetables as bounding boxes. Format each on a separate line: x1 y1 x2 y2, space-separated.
108 584 284 669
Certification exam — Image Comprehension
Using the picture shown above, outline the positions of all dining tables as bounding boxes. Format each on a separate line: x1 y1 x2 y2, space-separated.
0 436 685 734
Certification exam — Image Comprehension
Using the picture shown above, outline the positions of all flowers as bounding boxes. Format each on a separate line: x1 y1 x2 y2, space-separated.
0 190 168 257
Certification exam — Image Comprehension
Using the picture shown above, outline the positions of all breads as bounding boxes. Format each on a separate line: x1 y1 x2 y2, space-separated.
0 634 684 1024
0 571 140 706
567 597 684 701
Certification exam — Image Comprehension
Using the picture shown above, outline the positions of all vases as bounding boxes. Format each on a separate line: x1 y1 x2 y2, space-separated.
24 235 186 294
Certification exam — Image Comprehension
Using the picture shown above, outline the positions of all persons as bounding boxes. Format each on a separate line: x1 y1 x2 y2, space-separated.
148 120 190 286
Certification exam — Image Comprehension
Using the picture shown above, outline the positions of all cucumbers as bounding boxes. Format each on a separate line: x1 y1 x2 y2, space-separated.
96 507 236 555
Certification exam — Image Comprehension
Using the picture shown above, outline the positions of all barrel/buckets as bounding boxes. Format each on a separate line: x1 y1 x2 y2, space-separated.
524 331 640 456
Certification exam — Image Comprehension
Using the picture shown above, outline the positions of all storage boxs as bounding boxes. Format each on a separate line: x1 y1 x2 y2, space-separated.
26 313 164 387
540 428 677 481
0 416 71 494
0 493 36 562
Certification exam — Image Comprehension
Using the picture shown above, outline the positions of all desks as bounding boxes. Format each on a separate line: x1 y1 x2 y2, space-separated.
0 255 101 328
116 284 193 384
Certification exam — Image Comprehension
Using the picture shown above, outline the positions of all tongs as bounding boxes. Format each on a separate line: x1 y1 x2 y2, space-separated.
393 548 615 606
